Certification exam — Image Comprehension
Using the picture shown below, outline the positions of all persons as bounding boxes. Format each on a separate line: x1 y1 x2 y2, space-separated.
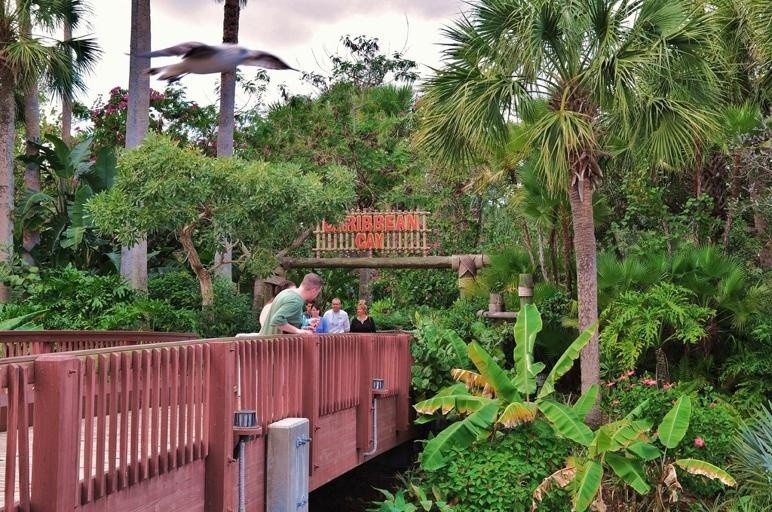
326 298 375 334
256 272 326 335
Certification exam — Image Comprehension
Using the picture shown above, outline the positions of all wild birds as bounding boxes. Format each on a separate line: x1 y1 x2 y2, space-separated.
123 41 299 81
